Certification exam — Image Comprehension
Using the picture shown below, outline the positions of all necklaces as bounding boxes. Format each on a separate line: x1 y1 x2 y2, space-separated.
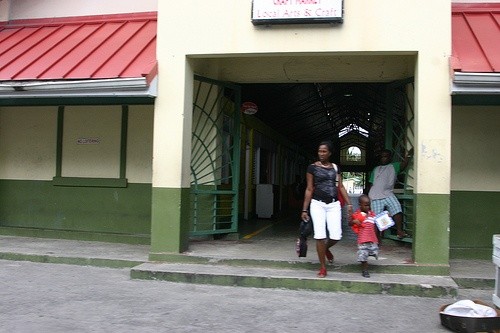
321 165 330 168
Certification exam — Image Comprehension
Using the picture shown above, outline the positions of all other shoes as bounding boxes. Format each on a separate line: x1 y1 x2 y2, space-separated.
362 271 370 277
318 268 327 278
325 249 333 263
397 233 408 239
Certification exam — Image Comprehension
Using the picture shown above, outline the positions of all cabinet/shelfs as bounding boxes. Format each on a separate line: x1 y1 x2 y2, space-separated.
255 183 280 218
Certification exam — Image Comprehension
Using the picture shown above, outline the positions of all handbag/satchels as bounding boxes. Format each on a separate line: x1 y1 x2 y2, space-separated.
337 186 345 207
296 219 313 257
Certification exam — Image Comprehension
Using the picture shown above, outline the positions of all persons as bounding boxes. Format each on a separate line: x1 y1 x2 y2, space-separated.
301 141 353 278
368 148 414 245
350 194 391 278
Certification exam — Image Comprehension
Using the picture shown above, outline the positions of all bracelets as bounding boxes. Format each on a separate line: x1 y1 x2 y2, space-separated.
302 211 307 212
408 156 411 158
347 204 352 205
